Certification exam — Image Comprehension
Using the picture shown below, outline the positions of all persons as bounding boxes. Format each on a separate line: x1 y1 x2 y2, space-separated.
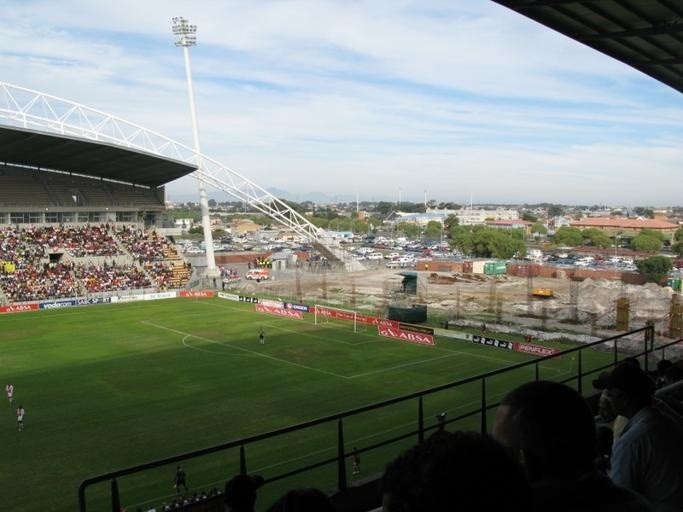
121 358 683 512
480 320 485 336
0 222 191 301
16 405 25 432
248 257 272 268
5 384 14 406
258 328 265 345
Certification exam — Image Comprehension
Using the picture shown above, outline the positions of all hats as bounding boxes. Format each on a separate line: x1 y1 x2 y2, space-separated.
592 366 656 394
224 474 265 497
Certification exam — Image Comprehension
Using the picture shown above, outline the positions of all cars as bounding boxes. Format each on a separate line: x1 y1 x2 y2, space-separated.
179 231 307 254
367 253 384 260
384 252 418 269
355 247 376 254
350 254 366 261
363 236 451 252
528 257 683 276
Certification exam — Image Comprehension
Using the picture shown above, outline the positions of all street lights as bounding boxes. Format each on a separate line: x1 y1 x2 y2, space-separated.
171 16 222 278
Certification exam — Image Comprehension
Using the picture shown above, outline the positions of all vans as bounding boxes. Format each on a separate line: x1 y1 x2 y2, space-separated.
245 269 270 280
